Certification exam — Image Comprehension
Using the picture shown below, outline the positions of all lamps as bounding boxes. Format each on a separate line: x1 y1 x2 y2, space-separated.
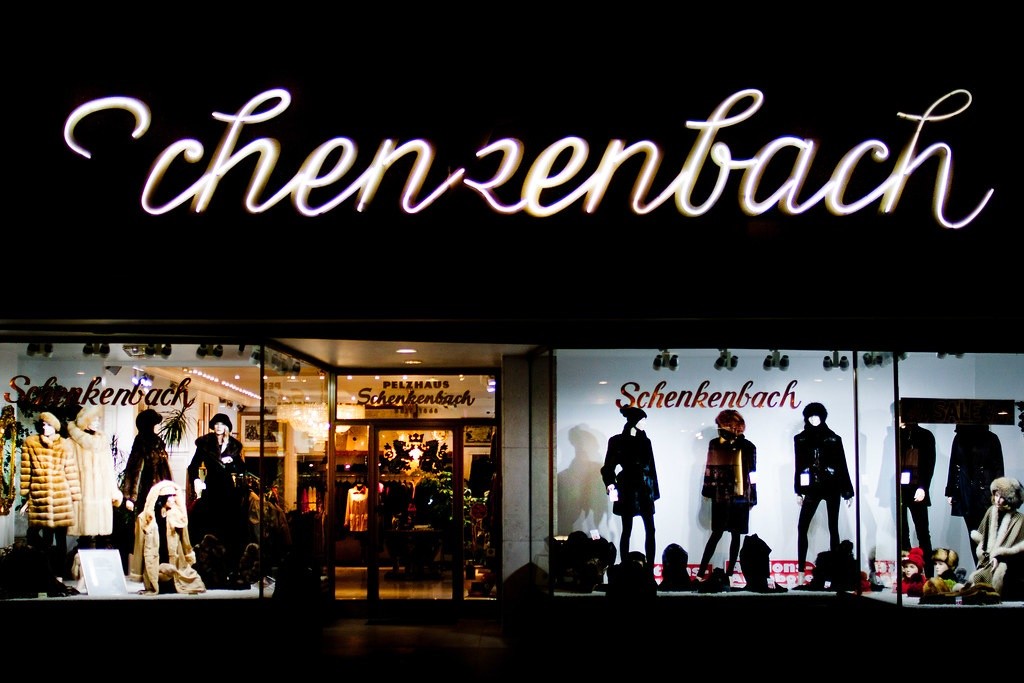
277 373 364 443
26 337 302 380
652 349 965 372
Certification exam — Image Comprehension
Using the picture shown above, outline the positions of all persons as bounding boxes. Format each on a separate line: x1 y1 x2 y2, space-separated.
876 407 937 585
900 546 929 594
794 402 854 586
969 476 1024 602
930 546 959 582
20 406 135 579
187 413 246 589
122 408 172 576
694 408 759 582
945 424 1005 567
601 404 661 578
130 480 207 596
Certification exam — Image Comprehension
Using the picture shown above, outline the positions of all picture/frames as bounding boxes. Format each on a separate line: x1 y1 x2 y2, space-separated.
464 424 497 447
236 410 287 453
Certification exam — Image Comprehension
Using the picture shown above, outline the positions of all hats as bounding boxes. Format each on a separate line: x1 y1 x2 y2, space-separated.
40 412 60 431
210 414 232 432
804 402 827 422
136 409 162 429
716 410 745 436
621 404 646 426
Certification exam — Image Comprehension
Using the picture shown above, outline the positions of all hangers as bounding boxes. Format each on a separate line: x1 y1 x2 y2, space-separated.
283 468 423 522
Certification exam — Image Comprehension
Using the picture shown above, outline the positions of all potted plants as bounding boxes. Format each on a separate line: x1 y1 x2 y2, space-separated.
428 470 487 556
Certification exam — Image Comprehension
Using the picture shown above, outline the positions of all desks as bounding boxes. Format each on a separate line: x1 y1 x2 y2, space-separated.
384 527 443 581
